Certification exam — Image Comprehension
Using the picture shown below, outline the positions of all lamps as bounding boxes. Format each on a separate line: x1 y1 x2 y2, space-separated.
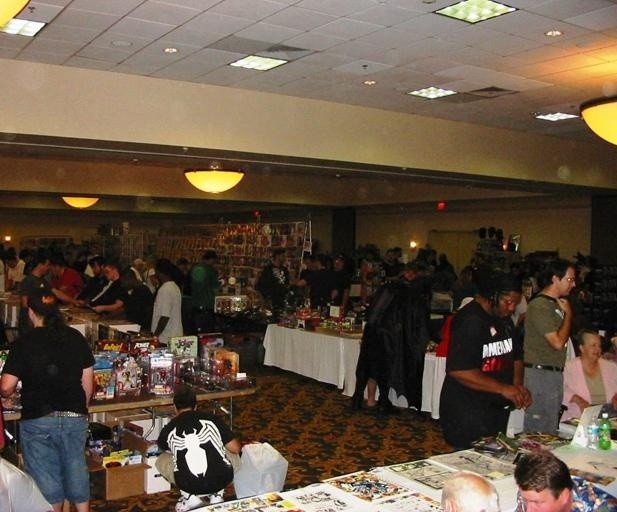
183 167 246 194
63 197 100 210
581 95 616 146
0 0 29 29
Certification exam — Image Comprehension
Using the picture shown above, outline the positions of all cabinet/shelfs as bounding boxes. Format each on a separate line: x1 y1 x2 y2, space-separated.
120 215 317 286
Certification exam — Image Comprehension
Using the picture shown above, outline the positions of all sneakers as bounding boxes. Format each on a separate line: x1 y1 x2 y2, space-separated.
174 495 204 512
378 397 392 408
351 393 364 405
209 489 224 504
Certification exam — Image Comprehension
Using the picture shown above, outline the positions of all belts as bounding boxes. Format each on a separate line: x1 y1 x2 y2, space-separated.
47 411 84 418
525 361 565 372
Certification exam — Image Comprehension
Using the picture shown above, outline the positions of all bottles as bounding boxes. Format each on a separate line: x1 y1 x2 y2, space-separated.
599 412 612 450
588 414 599 451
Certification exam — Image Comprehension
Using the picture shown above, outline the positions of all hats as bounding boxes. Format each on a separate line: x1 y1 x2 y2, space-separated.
273 249 285 256
334 253 347 261
134 259 145 264
28 289 58 314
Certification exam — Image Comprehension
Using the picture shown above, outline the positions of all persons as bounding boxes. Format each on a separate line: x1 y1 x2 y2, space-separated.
1 240 242 511
257 245 456 409
438 259 616 511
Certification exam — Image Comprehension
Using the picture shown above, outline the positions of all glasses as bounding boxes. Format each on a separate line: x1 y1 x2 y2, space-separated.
561 275 575 281
